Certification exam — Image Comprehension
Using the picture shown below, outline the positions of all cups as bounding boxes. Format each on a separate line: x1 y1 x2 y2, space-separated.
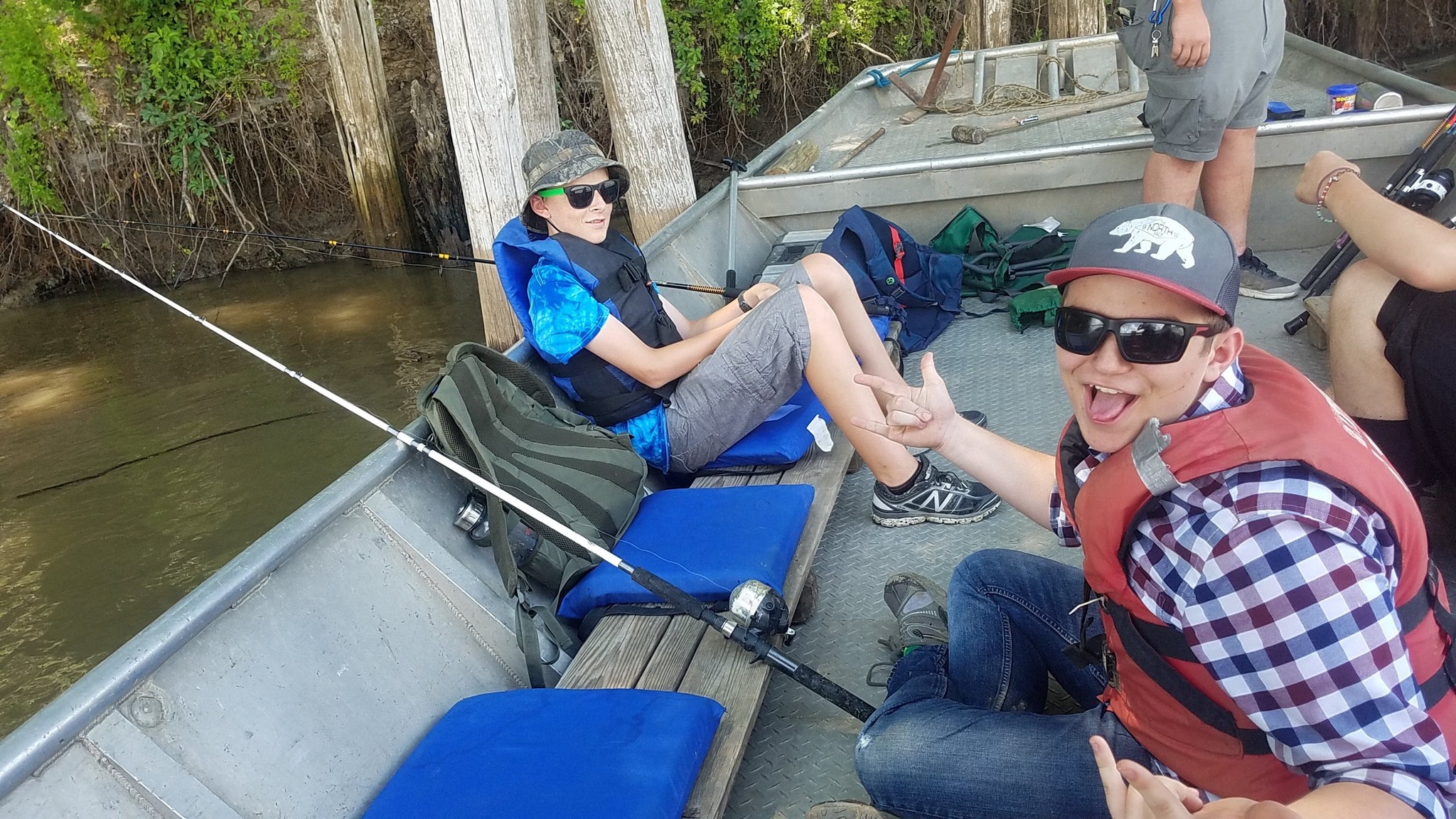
1325 84 1359 115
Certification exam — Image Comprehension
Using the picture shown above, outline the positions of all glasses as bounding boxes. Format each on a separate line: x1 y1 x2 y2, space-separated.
538 179 620 210
1052 306 1221 365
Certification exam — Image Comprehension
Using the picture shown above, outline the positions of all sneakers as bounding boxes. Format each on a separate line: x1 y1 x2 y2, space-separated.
866 572 952 687
1235 247 1301 300
870 454 1002 528
810 799 900 819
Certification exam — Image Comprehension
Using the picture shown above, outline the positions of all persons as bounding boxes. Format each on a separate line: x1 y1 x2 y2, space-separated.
1111 0 1302 301
1294 147 1456 564
492 128 1003 528
845 202 1456 819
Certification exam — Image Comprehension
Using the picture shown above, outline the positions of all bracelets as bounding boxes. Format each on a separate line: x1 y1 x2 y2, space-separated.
1316 168 1361 223
1316 167 1350 202
737 289 752 313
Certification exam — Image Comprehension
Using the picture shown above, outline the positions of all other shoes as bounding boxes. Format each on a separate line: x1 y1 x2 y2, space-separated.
957 410 989 429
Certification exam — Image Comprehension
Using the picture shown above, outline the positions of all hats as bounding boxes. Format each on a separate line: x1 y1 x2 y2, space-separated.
1045 202 1241 327
518 129 630 234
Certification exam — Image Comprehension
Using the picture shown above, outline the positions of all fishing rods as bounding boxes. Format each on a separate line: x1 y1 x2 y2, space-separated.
3 201 879 723
17 212 745 298
1283 104 1456 335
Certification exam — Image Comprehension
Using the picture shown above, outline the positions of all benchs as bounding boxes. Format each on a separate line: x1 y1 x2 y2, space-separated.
556 319 901 819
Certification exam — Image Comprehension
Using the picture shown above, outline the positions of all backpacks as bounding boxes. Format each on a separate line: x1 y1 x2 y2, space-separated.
820 204 966 355
420 342 650 688
